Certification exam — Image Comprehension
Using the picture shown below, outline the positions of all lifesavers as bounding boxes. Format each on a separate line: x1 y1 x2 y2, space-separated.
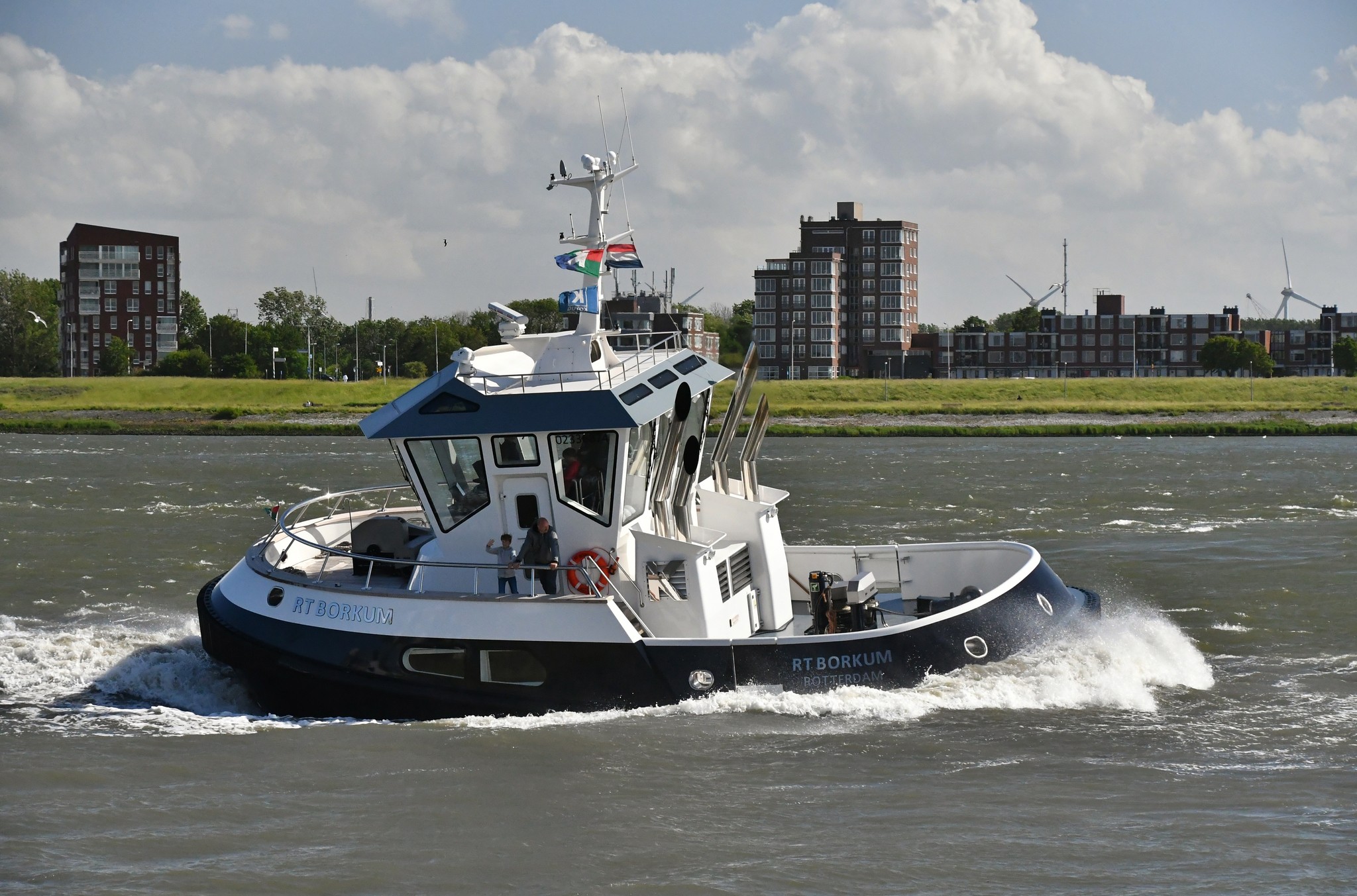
568 551 609 595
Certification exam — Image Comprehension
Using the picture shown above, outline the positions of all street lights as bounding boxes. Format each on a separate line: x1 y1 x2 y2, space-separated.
336 343 340 381
1064 362 1067 401
353 359 361 381
372 352 381 377
390 339 398 379
1055 361 1059 378
944 322 950 379
296 324 322 381
321 335 337 374
376 344 391 377
310 342 317 382
67 323 72 377
384 345 386 385
432 322 439 373
1250 361 1253 401
127 319 133 376
207 323 212 376
1328 316 1333 376
888 358 891 379
792 320 795 381
245 321 255 355
885 362 887 401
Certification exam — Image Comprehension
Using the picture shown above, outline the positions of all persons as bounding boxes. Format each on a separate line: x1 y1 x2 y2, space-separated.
485 533 520 594
513 518 560 594
501 436 520 460
562 448 581 496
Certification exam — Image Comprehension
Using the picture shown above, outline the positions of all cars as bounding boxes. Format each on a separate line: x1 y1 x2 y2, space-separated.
316 374 337 381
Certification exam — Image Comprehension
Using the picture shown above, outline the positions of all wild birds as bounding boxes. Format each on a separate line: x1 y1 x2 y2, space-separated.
1048 283 1065 294
1262 435 1267 438
1169 435 1173 438
26 309 48 329
1205 435 1215 438
1113 436 1122 440
443 239 449 247
1146 436 1151 439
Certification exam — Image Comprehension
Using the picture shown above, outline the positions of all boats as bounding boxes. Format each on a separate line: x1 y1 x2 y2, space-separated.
189 82 1109 720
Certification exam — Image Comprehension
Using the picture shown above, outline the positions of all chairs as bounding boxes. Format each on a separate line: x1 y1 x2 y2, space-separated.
568 465 594 504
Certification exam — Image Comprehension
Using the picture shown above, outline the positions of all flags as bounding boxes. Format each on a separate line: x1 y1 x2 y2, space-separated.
554 249 604 277
558 286 599 314
604 244 643 269
